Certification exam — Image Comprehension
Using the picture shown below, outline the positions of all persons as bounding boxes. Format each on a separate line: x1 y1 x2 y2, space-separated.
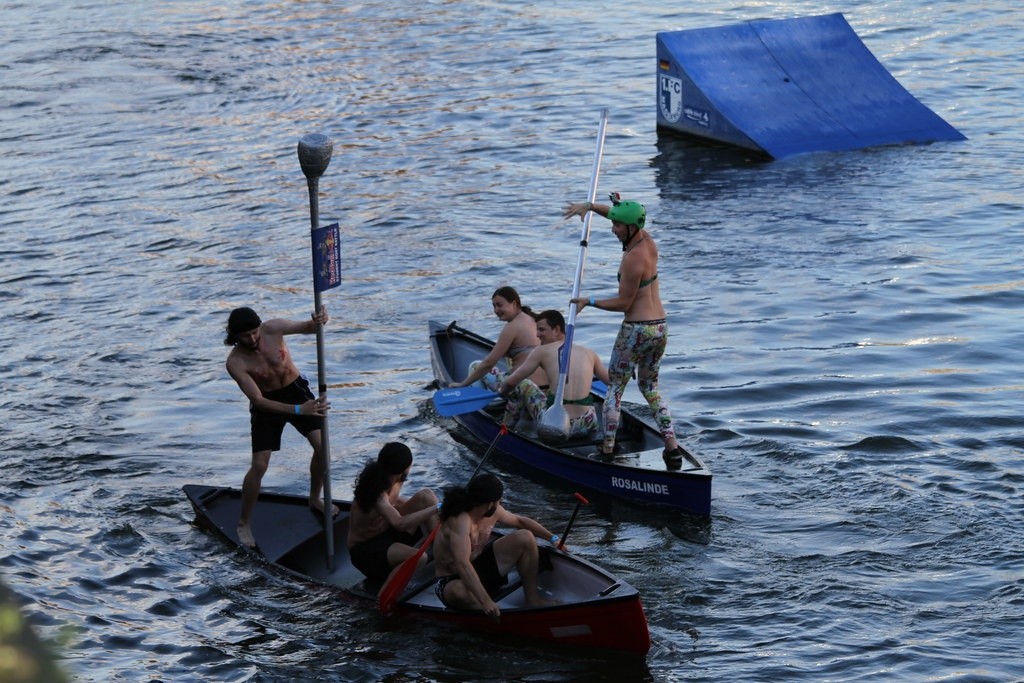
223 304 340 547
347 442 442 578
448 286 550 401
498 310 610 438
561 192 683 467
433 473 568 624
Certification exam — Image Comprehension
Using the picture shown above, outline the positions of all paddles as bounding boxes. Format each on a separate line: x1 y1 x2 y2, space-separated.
557 491 589 549
445 318 609 393
378 422 509 608
433 375 603 418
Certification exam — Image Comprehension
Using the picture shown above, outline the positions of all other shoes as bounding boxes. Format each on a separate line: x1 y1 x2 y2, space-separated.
588 452 616 464
662 449 682 470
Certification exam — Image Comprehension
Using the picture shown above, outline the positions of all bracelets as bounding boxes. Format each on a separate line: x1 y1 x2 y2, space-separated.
437 503 442 512
294 405 300 414
550 535 559 544
589 296 595 305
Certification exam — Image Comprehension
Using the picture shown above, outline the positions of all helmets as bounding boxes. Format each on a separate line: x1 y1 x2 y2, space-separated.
608 201 646 229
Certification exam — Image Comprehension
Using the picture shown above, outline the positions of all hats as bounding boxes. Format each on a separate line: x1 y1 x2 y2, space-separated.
377 442 412 474
229 307 262 336
468 474 503 503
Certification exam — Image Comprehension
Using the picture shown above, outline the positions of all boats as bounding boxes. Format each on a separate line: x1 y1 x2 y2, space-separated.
427 319 714 521
182 483 651 657
